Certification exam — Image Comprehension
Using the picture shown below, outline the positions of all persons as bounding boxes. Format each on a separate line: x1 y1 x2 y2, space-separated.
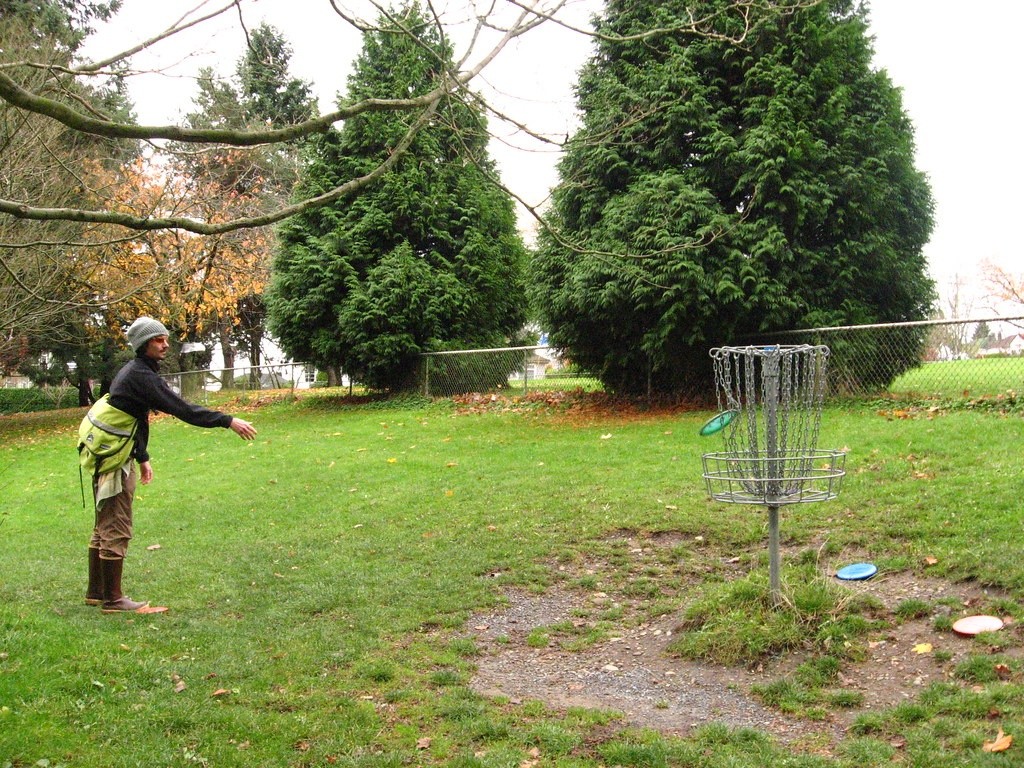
85 318 258 612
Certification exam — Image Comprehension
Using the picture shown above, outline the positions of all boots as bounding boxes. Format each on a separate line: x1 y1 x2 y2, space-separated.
85 546 105 605
98 553 149 613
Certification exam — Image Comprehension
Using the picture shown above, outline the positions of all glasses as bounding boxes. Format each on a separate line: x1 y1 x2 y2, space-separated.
151 336 168 343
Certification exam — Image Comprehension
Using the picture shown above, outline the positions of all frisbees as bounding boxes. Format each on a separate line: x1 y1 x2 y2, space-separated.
836 563 878 579
954 615 1004 637
698 409 741 436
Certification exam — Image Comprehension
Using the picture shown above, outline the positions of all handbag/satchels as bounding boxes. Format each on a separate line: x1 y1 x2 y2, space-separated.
77 392 138 476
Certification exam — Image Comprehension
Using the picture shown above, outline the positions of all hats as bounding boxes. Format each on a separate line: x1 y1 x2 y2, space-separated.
127 317 168 352
